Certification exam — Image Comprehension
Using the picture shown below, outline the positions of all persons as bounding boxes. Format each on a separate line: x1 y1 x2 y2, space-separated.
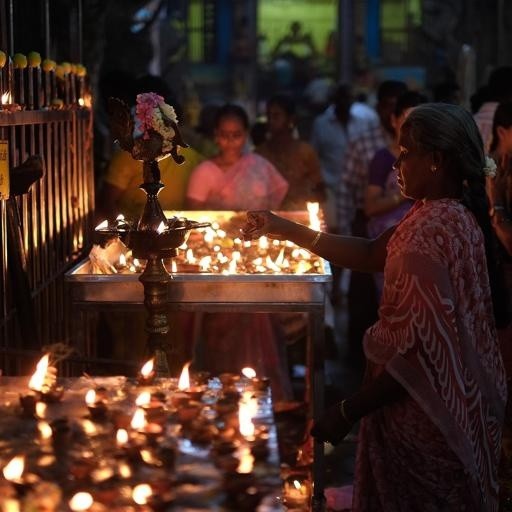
241 99 512 512
468 66 512 298
95 73 421 375
